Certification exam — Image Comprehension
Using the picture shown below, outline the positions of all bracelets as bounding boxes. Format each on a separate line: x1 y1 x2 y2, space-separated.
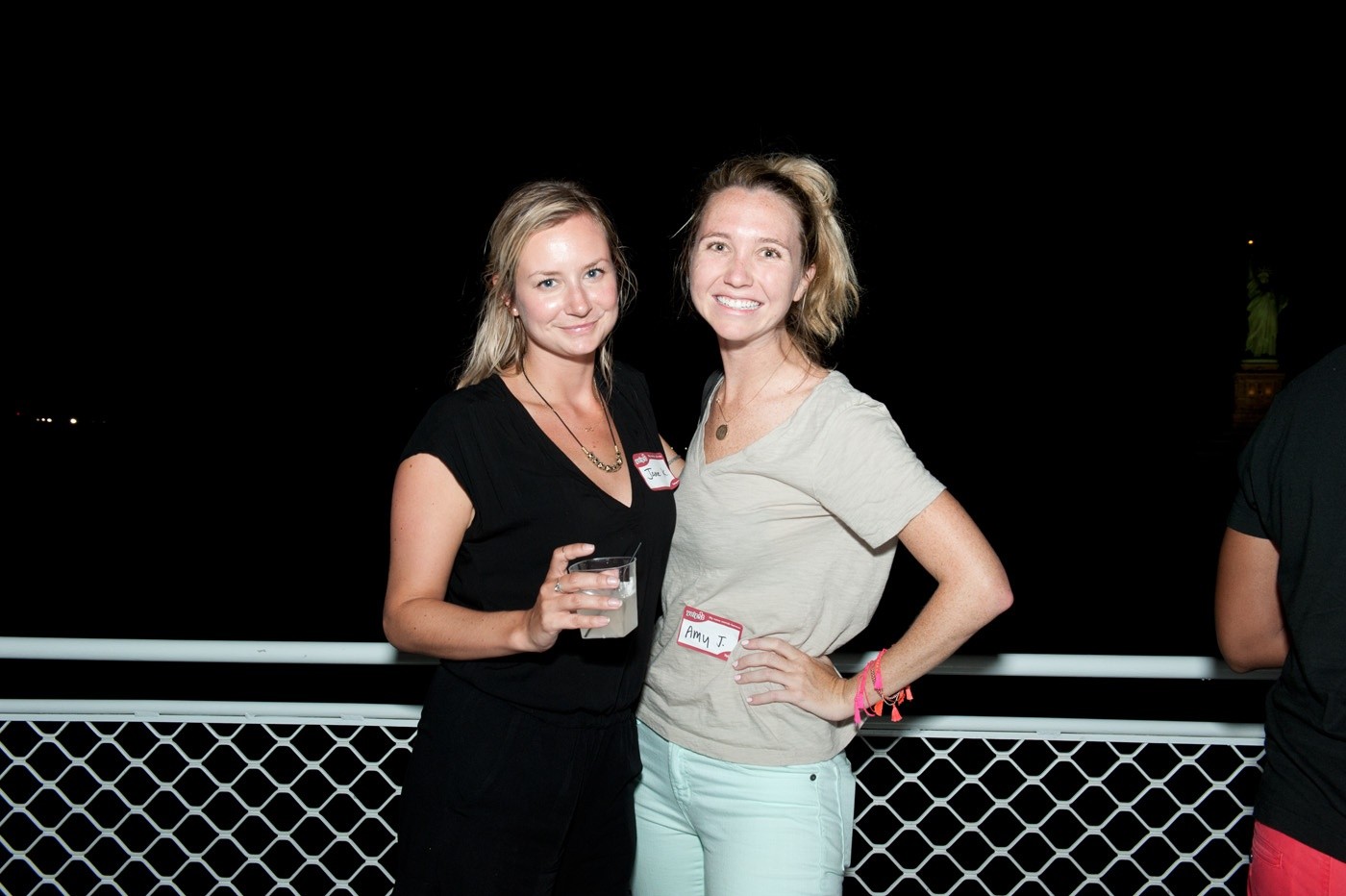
668 455 681 466
854 648 912 724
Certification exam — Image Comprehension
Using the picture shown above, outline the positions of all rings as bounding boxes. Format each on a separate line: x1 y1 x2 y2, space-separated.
555 578 567 594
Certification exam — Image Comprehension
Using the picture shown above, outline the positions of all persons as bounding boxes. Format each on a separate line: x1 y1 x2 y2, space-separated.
635 155 1013 896
1237 271 1286 408
381 155 1013 896
1215 315 1345 896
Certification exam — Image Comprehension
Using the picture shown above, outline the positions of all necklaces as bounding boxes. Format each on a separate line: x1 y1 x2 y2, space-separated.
714 342 793 440
521 356 624 472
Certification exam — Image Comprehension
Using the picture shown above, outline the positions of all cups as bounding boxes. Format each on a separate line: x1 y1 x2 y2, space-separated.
568 557 638 638
